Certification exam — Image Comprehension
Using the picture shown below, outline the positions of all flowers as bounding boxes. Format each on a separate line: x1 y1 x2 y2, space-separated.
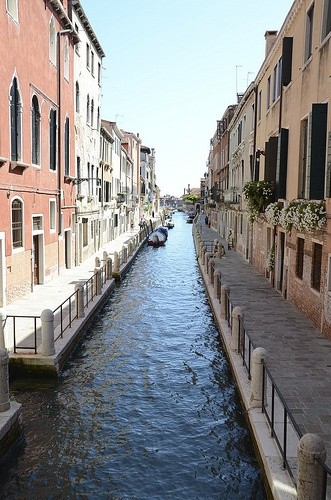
242 179 279 224
265 201 326 233
265 242 276 272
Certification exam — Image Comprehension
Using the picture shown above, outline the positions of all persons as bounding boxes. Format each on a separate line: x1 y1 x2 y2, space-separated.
141 214 148 229
139 217 144 231
205 215 208 225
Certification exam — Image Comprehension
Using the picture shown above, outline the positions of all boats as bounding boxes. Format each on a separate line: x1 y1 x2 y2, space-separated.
147 226 168 247
186 216 195 222
162 219 174 228
